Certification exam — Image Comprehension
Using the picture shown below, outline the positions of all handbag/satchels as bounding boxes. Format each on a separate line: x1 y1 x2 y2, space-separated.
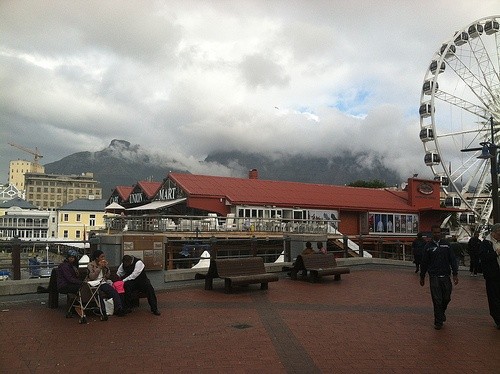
112 281 125 294
103 298 114 315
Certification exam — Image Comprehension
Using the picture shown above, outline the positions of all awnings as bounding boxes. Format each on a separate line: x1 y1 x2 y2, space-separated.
124 197 187 210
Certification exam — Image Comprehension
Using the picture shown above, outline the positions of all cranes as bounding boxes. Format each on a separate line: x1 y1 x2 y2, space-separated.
8 143 43 174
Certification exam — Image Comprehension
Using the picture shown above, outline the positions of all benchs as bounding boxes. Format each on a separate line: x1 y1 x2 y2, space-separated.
282 254 350 282
195 256 279 293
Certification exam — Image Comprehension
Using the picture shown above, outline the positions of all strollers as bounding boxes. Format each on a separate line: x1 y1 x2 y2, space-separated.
65 266 109 324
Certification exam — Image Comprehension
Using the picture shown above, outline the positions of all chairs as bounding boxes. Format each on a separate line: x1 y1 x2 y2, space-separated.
177 220 204 232
222 214 235 231
208 214 224 231
66 265 109 323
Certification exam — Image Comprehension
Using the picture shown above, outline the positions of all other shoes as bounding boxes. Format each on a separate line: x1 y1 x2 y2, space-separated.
434 313 446 328
287 273 292 275
415 269 419 273
75 306 84 317
151 308 161 315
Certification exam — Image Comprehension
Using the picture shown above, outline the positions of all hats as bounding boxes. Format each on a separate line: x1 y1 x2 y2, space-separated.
66 250 77 260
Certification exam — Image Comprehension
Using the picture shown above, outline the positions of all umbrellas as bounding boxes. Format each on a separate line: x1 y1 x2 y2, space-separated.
104 201 126 213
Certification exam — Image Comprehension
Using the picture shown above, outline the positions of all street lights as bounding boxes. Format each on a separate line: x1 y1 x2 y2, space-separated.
475 115 500 225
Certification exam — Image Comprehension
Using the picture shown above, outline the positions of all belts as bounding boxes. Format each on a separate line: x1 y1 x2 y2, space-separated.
432 275 448 277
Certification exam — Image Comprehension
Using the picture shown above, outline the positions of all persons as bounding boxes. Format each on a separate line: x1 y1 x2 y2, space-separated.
300 242 325 277
58 249 100 318
287 241 317 277
452 235 457 242
480 222 500 330
31 254 40 269
243 217 250 231
419 225 459 330
116 255 162 316
118 212 127 228
466 231 481 277
87 250 134 317
411 233 427 273
